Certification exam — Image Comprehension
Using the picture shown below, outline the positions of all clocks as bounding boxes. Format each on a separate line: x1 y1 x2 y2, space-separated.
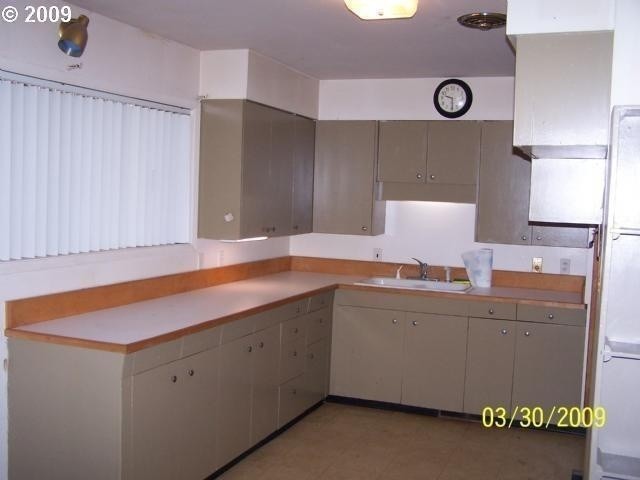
433 79 473 119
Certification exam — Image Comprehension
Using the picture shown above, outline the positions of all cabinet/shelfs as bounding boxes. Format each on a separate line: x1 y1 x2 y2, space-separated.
199 98 293 242
216 298 277 479
5 298 223 480
474 31 611 249
465 297 587 431
278 289 333 427
592 104 640 480
328 286 464 415
291 113 314 235
312 119 386 235
377 121 481 184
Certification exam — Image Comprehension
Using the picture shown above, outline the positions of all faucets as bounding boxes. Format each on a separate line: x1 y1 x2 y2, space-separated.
412 257 427 279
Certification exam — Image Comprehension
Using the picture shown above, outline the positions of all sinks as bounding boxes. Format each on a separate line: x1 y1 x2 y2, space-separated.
354 277 472 293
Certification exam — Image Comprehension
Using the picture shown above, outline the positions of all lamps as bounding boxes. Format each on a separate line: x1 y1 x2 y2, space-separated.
57 14 89 58
